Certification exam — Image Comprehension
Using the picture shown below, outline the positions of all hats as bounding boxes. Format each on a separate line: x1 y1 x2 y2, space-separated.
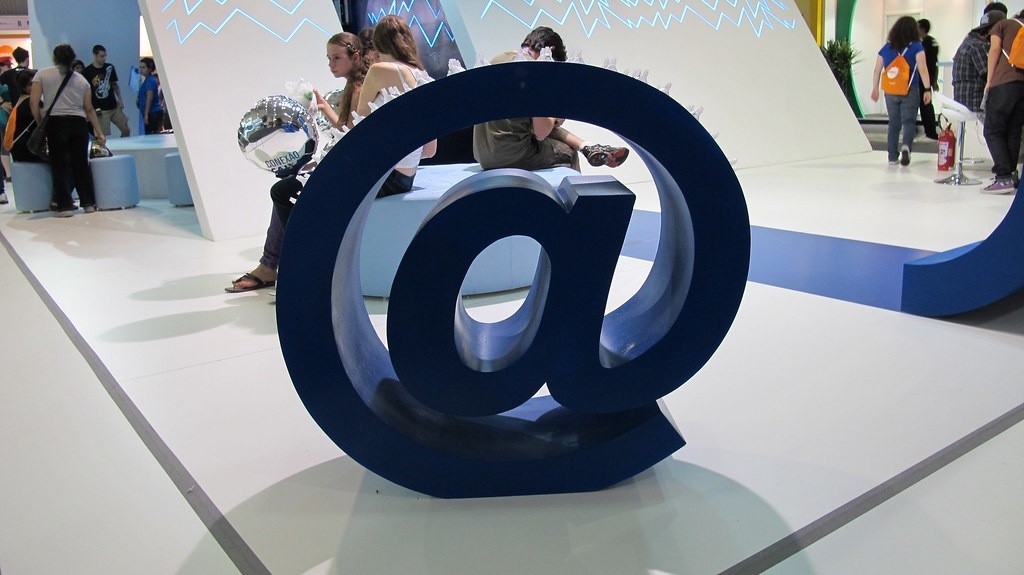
971 10 1005 30
12 47 29 63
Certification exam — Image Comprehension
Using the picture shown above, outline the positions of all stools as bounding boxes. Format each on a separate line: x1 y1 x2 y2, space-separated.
90 155 141 208
93 135 180 198
165 154 194 206
358 165 578 296
932 90 982 186
9 161 54 213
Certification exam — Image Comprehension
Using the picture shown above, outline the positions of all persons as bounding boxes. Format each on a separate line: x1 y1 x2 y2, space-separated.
80 45 131 138
71 60 98 140
136 57 174 134
915 19 939 140
951 2 1024 194
0 46 44 204
871 16 931 166
225 27 380 294
270 14 437 233
29 44 106 217
472 27 629 171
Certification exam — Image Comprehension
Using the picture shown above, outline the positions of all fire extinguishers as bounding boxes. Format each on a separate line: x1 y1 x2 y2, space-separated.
938 112 956 172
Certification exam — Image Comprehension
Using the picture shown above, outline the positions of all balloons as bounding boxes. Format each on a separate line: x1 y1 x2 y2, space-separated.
319 89 344 121
238 95 319 176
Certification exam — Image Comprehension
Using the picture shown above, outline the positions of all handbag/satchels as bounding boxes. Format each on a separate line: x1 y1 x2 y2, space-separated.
28 116 49 156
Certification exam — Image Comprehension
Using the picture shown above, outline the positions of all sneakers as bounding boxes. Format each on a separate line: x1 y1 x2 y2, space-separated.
581 144 629 168
980 177 1019 194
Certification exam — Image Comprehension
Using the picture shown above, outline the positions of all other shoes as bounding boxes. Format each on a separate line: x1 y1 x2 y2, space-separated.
900 145 911 165
85 206 95 212
889 160 900 167
1 193 8 203
55 209 74 217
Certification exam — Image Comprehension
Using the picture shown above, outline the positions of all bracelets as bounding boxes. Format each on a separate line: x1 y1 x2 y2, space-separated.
0 100 5 106
924 88 930 91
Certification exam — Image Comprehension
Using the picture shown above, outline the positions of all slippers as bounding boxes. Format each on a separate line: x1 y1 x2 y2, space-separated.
225 273 275 292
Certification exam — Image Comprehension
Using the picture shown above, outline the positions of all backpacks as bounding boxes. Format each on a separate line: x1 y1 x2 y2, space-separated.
3 95 35 151
881 41 917 96
1002 18 1024 69
